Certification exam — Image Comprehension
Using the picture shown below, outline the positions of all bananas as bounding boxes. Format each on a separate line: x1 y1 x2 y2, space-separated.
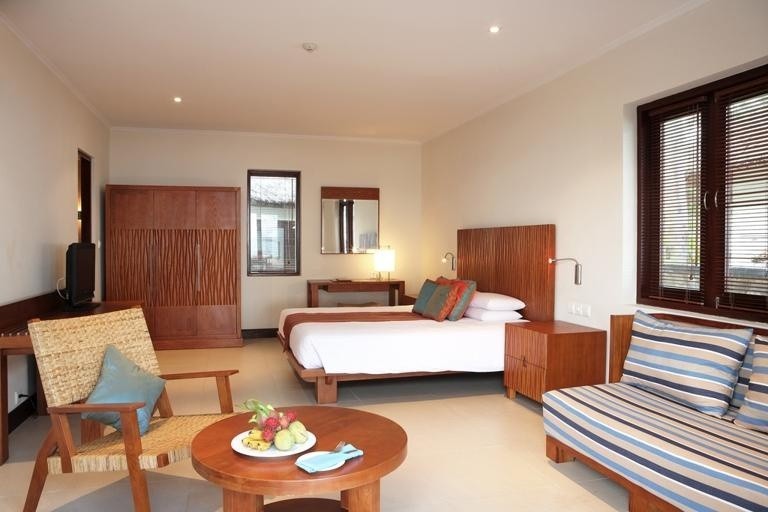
242 430 274 450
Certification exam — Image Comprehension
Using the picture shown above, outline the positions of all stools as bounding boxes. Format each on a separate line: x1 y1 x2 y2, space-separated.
336 299 387 308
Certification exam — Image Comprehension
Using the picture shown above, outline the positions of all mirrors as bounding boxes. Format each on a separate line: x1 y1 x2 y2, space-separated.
319 186 380 255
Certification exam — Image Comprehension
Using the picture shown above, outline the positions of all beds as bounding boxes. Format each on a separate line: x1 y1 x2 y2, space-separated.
276 222 557 406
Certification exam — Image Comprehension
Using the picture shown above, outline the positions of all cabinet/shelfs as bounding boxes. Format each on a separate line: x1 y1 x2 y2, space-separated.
100 182 151 328
193 184 244 350
150 184 198 350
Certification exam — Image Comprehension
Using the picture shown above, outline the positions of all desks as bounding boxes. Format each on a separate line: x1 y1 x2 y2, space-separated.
189 405 410 512
1 301 145 466
305 277 406 308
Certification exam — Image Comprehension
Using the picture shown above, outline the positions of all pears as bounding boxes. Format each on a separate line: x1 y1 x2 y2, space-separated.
274 421 309 451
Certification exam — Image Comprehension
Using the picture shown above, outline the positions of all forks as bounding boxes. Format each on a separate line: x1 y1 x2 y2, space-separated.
328 439 345 453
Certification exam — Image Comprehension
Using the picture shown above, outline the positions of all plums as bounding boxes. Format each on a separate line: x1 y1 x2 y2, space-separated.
262 410 297 441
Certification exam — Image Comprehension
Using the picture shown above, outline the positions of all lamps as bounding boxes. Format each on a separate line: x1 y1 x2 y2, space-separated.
441 252 455 271
373 244 395 280
547 257 581 286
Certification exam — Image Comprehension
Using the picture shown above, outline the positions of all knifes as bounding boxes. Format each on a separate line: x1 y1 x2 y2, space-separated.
343 449 358 456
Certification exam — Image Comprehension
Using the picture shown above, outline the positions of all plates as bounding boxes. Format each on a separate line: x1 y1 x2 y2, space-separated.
231 427 317 459
296 451 345 474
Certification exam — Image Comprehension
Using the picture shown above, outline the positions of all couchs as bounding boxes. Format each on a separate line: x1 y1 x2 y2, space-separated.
539 307 767 511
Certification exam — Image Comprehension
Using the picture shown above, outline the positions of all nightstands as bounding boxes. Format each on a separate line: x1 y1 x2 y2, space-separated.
400 293 418 305
500 319 607 404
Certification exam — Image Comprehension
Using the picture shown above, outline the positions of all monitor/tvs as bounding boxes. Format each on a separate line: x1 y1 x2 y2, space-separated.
65 243 101 311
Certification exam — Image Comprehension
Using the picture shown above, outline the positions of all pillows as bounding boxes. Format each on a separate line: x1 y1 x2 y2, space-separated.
467 289 527 311
435 274 477 321
77 341 168 437
462 306 525 322
409 277 459 321
618 306 755 422
732 328 766 434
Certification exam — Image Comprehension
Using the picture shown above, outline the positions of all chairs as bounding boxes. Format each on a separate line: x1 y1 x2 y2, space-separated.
22 301 246 512
